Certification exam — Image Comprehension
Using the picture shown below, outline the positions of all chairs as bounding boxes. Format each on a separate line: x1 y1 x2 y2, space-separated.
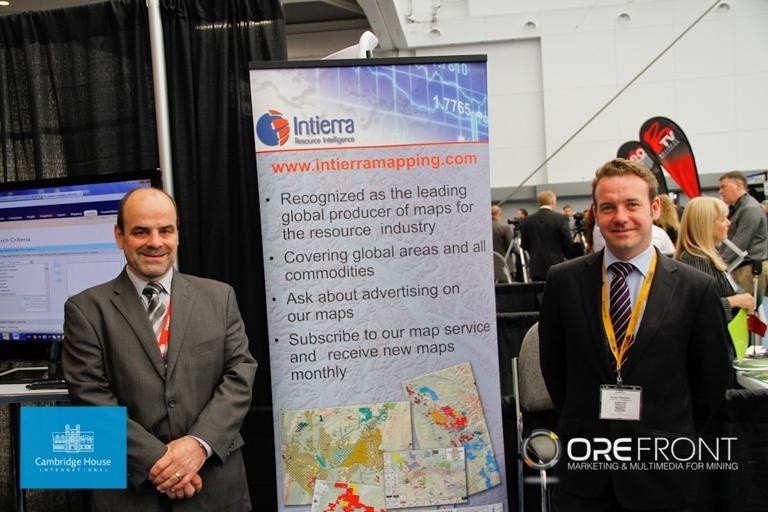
509 317 561 512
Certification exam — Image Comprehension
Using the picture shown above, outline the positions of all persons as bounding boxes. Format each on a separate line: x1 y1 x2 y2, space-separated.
62 186 257 512
537 157 736 512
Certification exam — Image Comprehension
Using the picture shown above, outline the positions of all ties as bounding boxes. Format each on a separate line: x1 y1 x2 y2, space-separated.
605 261 636 375
141 280 173 371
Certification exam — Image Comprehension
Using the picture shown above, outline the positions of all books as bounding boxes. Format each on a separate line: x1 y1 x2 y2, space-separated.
727 308 751 364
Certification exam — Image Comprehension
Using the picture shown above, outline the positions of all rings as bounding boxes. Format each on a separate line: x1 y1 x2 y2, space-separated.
175 473 181 480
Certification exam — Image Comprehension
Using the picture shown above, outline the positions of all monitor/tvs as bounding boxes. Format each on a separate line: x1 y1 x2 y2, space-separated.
0 169 164 361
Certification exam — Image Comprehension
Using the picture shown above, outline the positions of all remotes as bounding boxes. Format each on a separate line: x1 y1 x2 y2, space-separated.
26 379 68 390
